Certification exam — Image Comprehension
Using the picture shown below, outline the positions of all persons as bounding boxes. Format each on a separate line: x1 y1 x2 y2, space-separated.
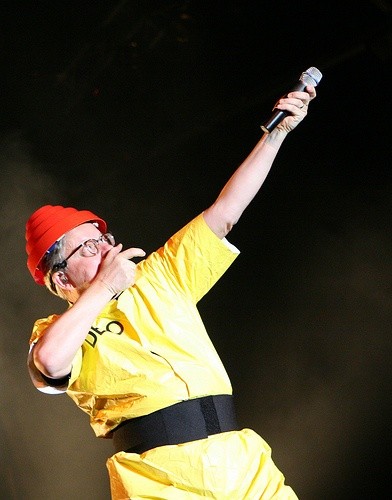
25 84 316 500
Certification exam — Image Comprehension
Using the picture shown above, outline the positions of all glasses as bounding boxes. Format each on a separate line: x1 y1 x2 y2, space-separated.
50 233 115 295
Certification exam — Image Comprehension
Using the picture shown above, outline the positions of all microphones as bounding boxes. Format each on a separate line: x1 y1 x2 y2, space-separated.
260 67 322 134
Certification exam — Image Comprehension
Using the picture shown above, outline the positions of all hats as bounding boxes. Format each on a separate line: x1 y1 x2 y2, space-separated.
25 205 107 287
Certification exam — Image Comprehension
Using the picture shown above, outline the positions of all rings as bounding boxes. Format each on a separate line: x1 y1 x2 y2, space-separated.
300 104 304 109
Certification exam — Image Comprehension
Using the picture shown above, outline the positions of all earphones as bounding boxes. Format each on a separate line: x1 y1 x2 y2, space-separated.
61 279 70 284
